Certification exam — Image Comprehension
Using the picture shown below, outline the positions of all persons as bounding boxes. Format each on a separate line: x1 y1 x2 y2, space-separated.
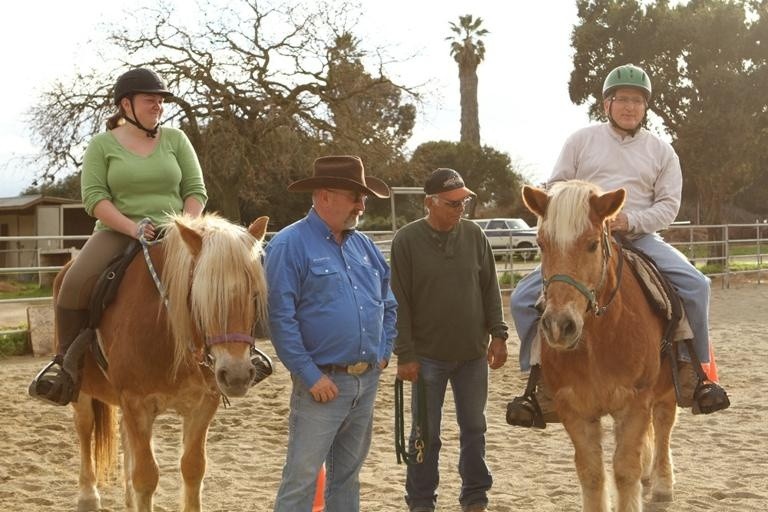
264 155 400 512
38 69 271 405
391 168 508 512
507 64 724 427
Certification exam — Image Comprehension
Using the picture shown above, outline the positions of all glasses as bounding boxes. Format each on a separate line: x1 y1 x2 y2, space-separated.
605 96 646 104
433 196 472 208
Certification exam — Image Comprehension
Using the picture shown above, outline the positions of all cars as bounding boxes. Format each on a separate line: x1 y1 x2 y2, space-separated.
469 218 540 261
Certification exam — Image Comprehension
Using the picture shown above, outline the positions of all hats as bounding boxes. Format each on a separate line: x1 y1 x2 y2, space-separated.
424 168 477 202
287 156 391 199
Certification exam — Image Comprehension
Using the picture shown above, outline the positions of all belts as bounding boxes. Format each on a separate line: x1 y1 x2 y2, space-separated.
318 363 375 373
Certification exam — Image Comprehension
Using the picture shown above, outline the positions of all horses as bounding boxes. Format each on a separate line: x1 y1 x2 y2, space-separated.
52 201 272 512
521 178 678 512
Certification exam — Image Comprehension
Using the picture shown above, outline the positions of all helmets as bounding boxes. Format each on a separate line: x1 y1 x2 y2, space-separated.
602 63 652 101
114 69 173 104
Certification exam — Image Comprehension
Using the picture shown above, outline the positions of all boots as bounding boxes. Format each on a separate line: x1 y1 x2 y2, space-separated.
678 361 723 407
36 306 89 401
249 360 269 384
508 365 562 423
